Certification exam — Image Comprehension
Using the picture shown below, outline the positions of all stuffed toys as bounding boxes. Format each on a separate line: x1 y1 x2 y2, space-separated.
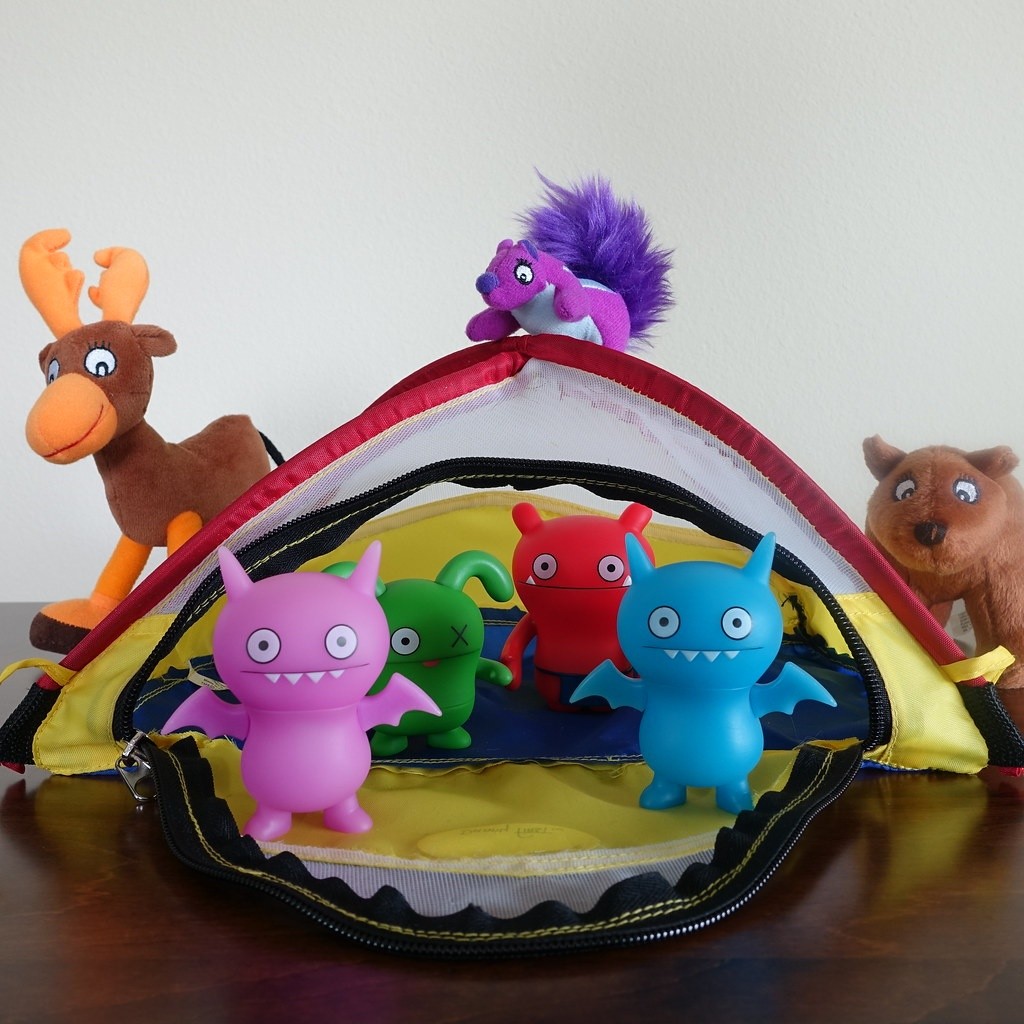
861 434 1023 693
19 230 286 657
466 166 676 355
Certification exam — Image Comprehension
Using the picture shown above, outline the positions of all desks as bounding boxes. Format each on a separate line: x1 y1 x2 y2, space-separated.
0 600 1024 1024
